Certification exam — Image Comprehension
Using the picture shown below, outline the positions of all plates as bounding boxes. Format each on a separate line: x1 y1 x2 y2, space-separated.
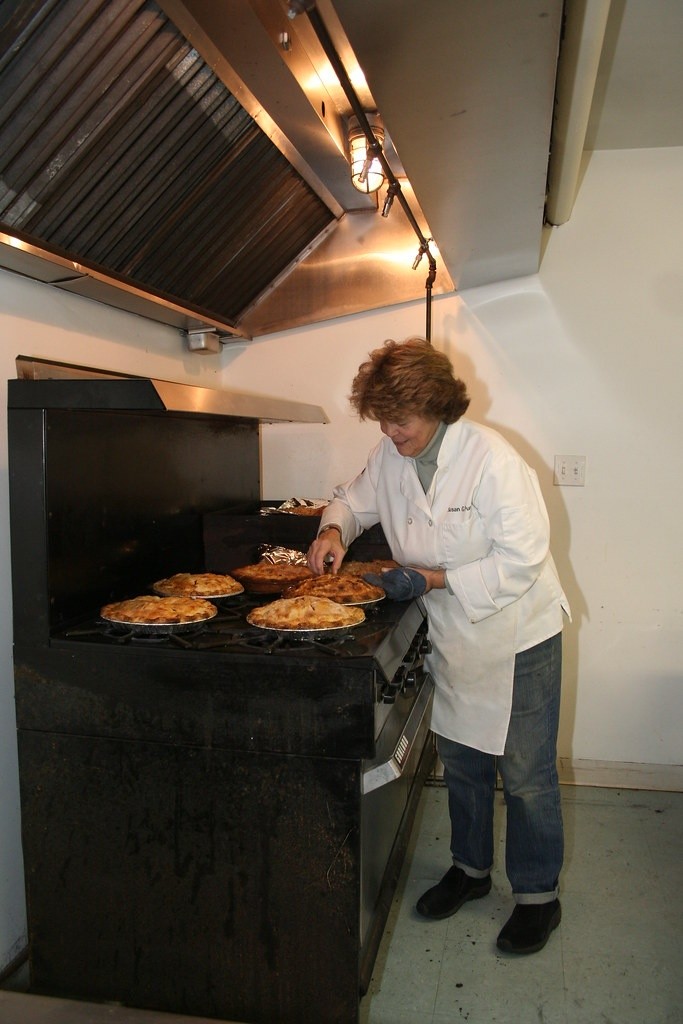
247 605 366 632
277 587 387 607
100 610 218 626
155 584 245 599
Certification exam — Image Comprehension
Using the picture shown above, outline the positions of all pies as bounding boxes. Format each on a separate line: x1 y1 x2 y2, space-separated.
230 560 403 631
153 572 243 596
99 595 216 624
284 506 328 516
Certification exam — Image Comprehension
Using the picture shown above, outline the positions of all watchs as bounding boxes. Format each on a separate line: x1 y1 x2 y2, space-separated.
318 525 340 539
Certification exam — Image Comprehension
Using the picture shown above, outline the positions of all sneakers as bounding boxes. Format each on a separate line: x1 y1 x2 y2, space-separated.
417 865 492 919
496 897 562 954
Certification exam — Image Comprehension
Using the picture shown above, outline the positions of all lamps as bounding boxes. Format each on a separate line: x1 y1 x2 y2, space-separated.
349 113 386 194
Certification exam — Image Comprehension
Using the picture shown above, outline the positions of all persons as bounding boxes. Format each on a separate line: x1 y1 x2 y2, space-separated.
308 336 574 959
